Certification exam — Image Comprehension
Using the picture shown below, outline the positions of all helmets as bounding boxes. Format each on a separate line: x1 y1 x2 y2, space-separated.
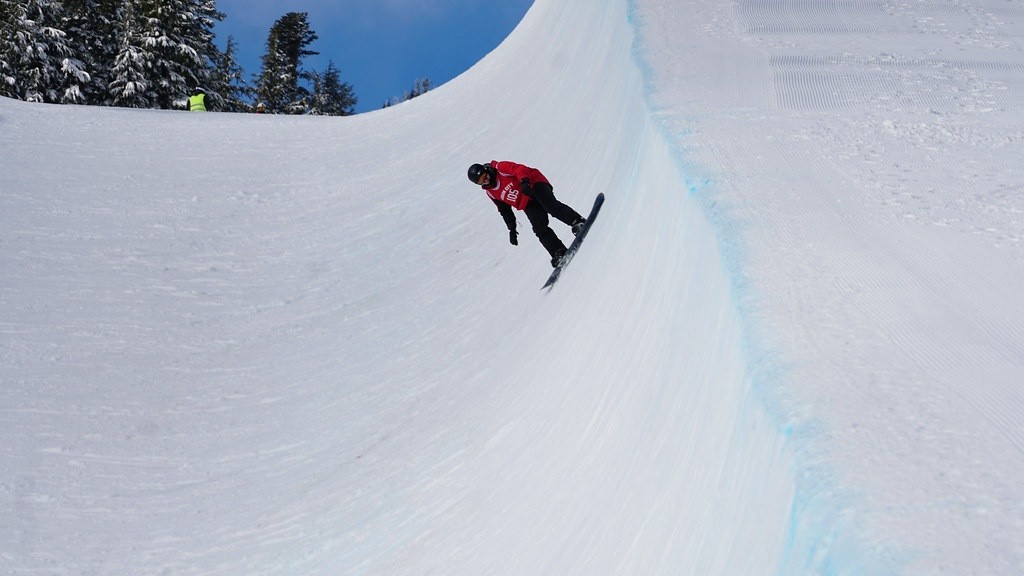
468 164 485 184
257 103 266 108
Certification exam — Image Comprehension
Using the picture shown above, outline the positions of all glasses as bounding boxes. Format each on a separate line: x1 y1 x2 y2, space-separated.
478 172 490 185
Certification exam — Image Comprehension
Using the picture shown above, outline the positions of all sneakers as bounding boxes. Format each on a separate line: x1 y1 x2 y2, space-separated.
551 247 568 268
571 217 587 236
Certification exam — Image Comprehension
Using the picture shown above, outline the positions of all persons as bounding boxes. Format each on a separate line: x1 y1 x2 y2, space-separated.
186 87 213 112
467 160 586 269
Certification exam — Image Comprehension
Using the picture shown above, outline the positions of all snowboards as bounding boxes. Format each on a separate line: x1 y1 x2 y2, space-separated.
539 191 605 291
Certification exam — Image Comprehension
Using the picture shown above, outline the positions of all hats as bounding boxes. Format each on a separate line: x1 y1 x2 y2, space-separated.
195 84 206 92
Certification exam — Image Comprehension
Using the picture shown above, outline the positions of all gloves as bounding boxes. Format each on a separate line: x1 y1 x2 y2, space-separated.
520 177 531 194
510 229 519 246
